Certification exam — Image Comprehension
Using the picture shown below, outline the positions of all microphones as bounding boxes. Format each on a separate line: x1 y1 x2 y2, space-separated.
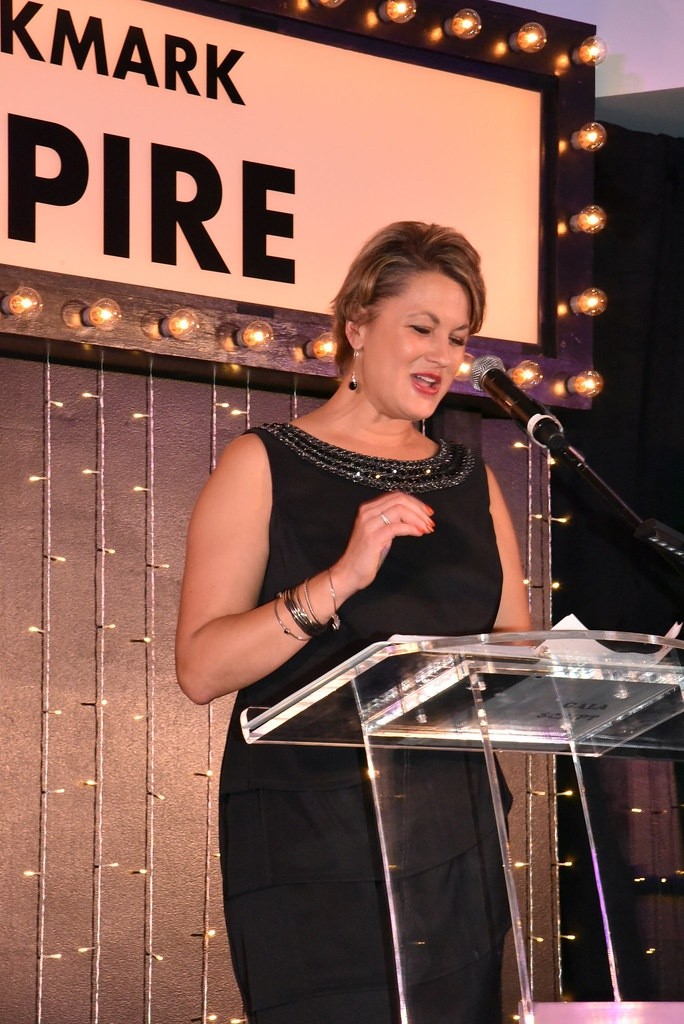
470 355 564 449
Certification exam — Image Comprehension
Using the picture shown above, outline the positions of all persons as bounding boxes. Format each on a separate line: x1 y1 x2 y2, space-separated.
174 222 530 1024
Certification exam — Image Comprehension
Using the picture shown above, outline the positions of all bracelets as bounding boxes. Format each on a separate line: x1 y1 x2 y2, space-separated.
275 567 341 641
